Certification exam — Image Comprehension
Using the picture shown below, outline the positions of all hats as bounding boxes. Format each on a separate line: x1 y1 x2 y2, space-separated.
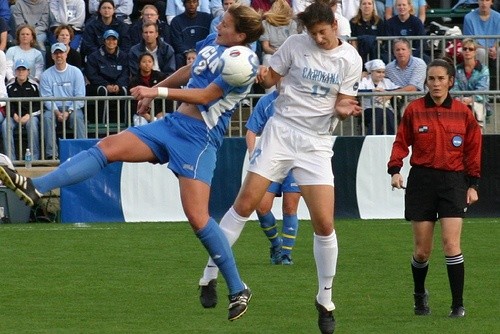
365 59 386 73
13 58 30 70
51 43 67 54
103 29 119 40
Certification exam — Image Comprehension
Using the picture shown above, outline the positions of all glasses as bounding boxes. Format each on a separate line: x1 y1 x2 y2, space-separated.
463 47 476 52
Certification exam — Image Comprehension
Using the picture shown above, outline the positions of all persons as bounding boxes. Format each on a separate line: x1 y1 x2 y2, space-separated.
387 57 482 318
199 1 363 334
450 38 490 125
0 0 292 321
463 0 500 66
245 84 301 264
0 0 430 161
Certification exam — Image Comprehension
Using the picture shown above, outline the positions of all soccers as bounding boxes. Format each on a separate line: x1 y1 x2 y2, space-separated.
218 46 260 85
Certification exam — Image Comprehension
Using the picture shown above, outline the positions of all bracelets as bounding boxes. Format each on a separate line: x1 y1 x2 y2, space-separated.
157 87 169 99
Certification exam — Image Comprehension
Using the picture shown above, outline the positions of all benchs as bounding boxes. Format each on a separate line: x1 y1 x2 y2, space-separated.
425 7 475 18
11 123 132 135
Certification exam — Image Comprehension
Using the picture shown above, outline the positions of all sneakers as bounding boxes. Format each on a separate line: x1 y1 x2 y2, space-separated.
0 164 44 206
228 281 253 322
198 278 218 308
281 252 295 266
414 288 430 316
314 295 336 334
242 99 251 107
449 303 465 317
270 238 283 265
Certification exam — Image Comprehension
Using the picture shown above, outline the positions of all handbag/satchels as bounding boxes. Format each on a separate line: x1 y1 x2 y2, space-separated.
425 19 463 49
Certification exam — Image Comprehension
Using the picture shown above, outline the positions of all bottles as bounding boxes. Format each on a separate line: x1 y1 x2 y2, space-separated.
25 148 32 168
